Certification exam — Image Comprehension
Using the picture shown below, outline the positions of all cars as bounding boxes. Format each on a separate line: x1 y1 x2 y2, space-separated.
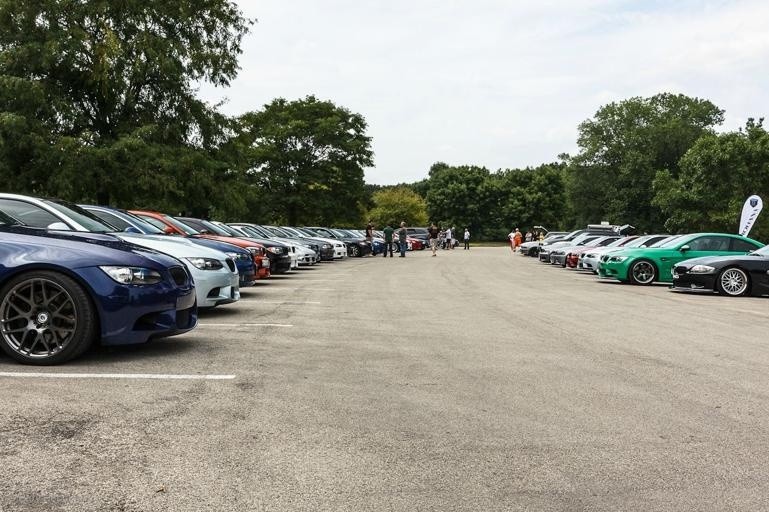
513 222 769 297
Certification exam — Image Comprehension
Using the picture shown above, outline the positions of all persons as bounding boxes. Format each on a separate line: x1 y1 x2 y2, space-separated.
382 223 396 257
365 220 374 256
398 222 407 257
463 228 471 250
508 228 545 252
427 222 456 256
